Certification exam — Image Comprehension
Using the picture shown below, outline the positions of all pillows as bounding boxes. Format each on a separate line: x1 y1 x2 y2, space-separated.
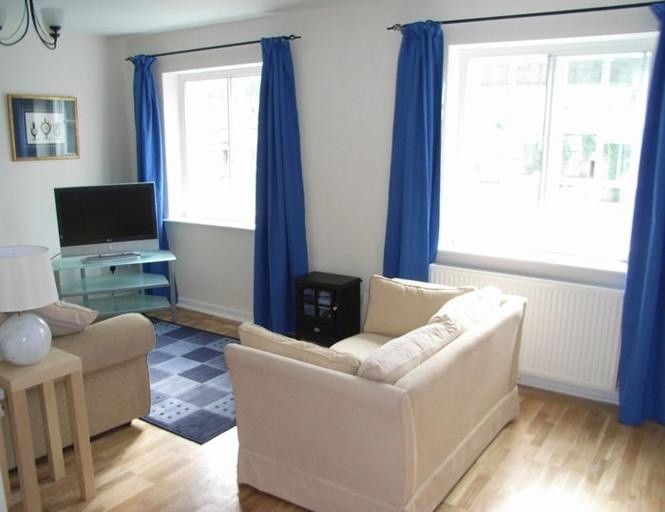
427 286 502 337
239 321 361 376
356 321 460 385
35 300 99 338
362 273 475 336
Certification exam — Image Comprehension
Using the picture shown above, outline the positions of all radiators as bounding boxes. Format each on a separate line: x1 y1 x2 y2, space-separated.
427 263 626 394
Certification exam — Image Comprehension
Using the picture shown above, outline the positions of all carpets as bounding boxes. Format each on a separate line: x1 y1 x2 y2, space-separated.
141 312 241 445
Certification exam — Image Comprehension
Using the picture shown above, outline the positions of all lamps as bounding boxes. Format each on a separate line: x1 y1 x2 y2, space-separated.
0 0 65 50
0 245 58 365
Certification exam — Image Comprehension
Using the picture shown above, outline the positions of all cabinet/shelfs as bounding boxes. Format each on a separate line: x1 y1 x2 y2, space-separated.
53 250 177 322
294 272 362 347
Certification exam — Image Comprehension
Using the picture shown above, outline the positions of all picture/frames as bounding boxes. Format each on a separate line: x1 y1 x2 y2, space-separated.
7 94 81 161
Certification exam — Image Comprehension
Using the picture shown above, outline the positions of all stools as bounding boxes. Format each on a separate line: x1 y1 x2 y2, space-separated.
0 347 96 512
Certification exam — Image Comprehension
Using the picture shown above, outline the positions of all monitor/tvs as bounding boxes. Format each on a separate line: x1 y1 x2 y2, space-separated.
54 181 159 264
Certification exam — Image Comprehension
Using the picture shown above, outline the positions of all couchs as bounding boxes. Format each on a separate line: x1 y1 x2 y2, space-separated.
225 277 528 512
0 312 156 471
